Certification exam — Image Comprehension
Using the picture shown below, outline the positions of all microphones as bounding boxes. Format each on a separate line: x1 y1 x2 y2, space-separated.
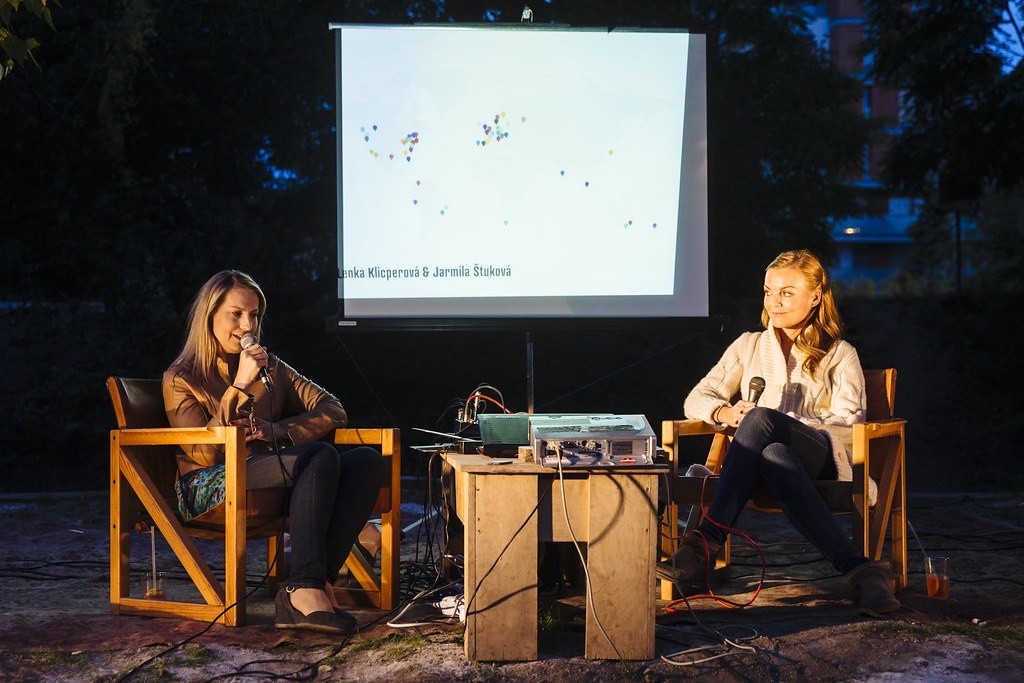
241 333 271 394
748 377 766 404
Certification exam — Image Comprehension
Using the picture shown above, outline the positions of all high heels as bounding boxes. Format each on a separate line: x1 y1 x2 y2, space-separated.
275 578 357 633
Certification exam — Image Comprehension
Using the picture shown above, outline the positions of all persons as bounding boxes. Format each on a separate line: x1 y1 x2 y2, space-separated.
161 271 385 634
657 250 900 616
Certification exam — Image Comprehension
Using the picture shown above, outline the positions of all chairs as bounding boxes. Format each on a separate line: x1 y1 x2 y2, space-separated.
664 367 910 601
105 378 402 625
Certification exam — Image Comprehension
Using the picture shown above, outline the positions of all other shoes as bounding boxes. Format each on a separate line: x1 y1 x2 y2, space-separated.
656 532 721 589
847 561 900 613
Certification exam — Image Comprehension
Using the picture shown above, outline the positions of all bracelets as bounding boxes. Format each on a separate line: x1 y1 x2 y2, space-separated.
716 405 728 424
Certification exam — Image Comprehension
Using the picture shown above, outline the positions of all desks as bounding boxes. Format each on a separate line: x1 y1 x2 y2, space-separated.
439 453 669 660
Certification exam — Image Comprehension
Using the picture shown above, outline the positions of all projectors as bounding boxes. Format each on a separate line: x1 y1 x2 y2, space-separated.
526 415 657 468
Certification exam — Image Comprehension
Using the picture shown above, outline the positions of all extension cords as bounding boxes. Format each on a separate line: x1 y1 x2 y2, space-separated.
441 596 465 615
